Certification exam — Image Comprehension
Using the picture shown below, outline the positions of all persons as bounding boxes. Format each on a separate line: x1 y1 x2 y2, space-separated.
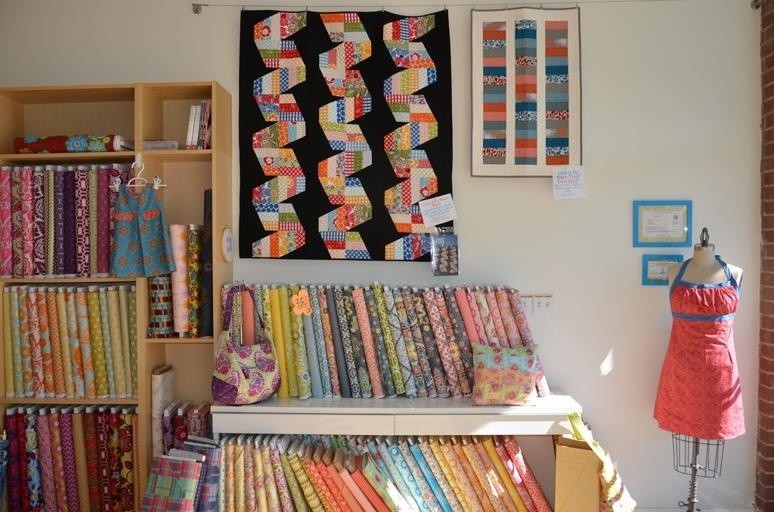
650 238 749 440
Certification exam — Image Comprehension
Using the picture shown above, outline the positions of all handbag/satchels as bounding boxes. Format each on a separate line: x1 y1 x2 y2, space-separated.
212 285 280 404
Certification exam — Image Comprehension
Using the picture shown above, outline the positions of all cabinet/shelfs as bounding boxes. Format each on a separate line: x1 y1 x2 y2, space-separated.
208 387 585 510
2 77 236 510
